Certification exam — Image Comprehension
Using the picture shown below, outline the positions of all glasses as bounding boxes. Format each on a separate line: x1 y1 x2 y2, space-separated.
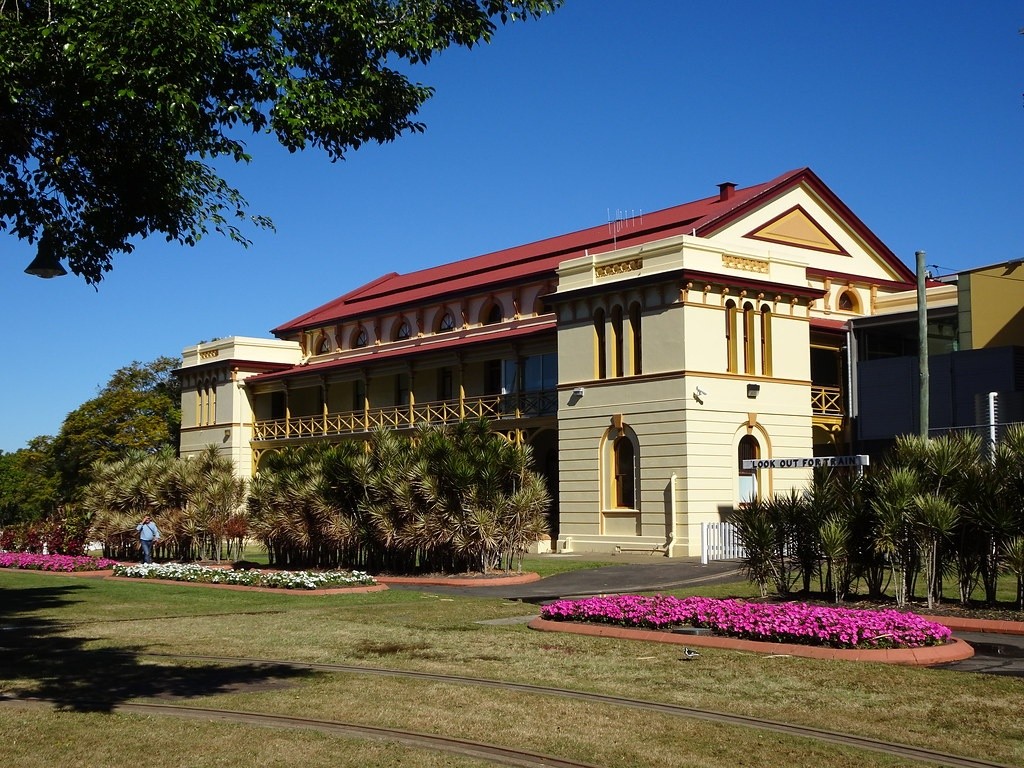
147 518 150 519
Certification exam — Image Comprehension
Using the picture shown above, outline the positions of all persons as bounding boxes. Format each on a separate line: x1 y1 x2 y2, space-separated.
136 514 159 564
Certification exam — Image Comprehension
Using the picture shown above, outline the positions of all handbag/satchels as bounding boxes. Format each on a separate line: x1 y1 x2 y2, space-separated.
153 534 157 542
